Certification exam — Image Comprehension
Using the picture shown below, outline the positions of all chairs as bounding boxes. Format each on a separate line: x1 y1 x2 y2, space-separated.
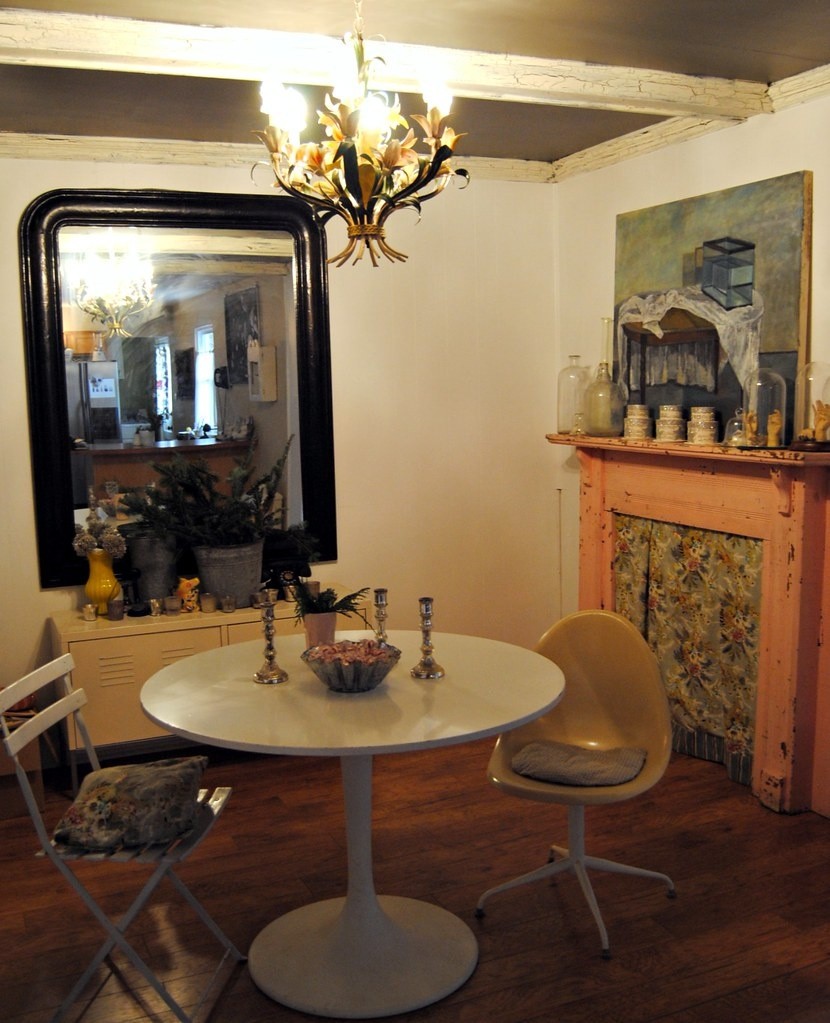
474 609 676 961
0 652 250 1022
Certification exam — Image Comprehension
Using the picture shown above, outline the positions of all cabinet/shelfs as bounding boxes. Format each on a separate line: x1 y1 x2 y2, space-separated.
49 600 374 800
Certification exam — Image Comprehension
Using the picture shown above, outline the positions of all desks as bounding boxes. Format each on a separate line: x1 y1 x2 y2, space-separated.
139 628 567 1020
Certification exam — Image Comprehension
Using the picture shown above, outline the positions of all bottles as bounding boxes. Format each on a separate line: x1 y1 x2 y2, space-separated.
584 362 624 437
557 354 592 435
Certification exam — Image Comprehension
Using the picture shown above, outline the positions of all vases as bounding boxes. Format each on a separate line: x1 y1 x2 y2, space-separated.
85 548 120 615
125 525 177 608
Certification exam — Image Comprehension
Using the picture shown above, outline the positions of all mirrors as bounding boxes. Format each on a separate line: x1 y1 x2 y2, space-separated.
16 186 339 587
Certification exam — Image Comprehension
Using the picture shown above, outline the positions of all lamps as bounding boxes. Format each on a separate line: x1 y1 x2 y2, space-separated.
74 230 158 339
252 0 469 269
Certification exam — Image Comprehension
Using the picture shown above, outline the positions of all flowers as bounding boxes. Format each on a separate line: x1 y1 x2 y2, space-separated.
74 523 127 559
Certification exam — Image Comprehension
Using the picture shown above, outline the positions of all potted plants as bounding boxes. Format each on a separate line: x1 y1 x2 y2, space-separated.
287 574 376 650
120 432 295 607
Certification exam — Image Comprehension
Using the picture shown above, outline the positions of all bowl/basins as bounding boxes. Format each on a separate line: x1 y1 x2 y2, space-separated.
300 640 401 693
